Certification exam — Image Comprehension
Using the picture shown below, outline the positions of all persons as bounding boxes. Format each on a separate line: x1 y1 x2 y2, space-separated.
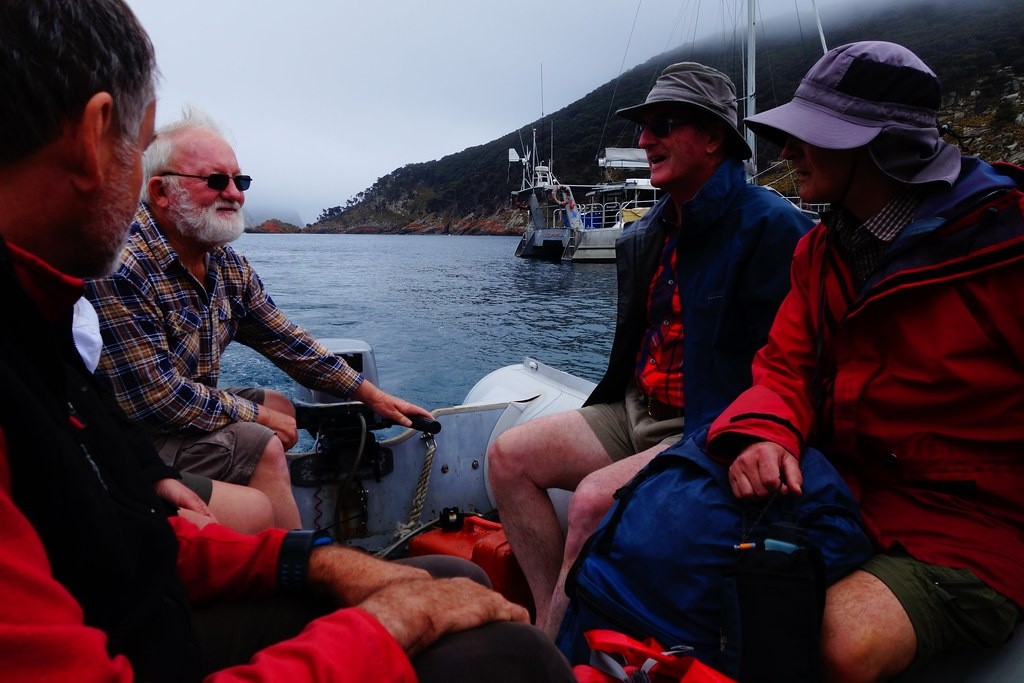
487 42 1024 683
0 0 576 683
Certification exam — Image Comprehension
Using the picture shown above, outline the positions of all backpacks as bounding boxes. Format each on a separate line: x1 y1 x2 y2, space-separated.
553 422 876 683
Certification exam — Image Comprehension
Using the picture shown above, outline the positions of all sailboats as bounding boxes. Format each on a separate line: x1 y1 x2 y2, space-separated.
509 0 832 266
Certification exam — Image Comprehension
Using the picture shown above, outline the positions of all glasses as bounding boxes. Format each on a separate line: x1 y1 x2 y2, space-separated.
159 172 253 191
640 113 709 137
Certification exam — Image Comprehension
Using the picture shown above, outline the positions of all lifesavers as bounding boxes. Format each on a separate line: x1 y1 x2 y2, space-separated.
552 186 572 205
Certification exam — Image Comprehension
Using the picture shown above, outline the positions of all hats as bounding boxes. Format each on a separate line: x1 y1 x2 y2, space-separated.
742 40 943 149
615 61 751 161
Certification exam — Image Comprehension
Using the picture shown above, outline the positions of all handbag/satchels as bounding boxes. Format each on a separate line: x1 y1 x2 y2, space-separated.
716 479 825 683
